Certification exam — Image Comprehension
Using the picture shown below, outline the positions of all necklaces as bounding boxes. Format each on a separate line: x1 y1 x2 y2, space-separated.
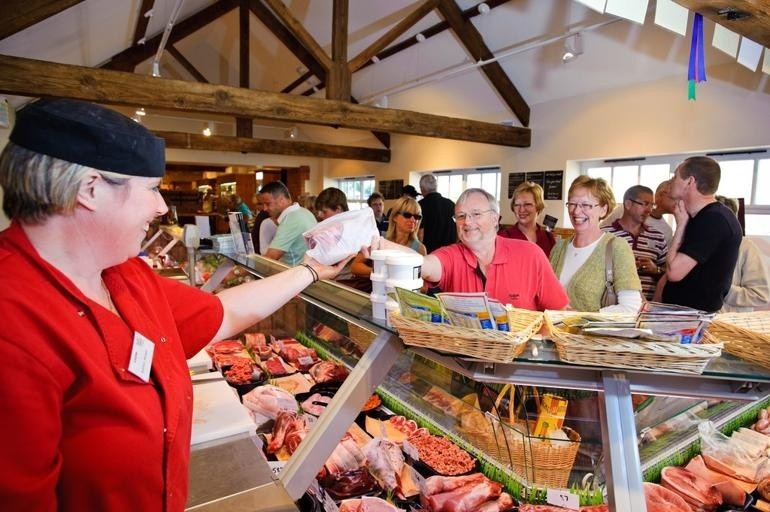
101 279 112 312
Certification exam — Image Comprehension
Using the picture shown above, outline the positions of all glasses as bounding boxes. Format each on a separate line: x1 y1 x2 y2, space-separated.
564 199 602 212
396 209 423 222
627 196 657 210
450 209 494 225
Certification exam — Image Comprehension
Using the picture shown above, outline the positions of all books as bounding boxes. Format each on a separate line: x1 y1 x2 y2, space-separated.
207 232 235 250
227 212 250 255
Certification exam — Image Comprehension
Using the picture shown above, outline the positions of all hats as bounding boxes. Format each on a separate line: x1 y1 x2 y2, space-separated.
7 96 166 179
397 184 422 198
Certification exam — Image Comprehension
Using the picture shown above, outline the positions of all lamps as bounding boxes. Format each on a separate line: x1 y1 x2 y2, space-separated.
132 117 142 123
289 130 296 138
560 33 584 65
202 128 211 138
135 109 146 116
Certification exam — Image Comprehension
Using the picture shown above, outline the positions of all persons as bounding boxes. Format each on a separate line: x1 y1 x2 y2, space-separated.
1 93 356 511
315 188 349 221
250 181 317 266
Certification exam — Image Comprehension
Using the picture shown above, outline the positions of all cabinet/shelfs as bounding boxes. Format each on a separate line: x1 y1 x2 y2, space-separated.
191 179 216 214
169 180 191 191
215 174 256 233
138 225 770 512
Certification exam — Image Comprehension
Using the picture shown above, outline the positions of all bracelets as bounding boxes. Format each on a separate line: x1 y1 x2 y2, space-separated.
300 262 319 283
